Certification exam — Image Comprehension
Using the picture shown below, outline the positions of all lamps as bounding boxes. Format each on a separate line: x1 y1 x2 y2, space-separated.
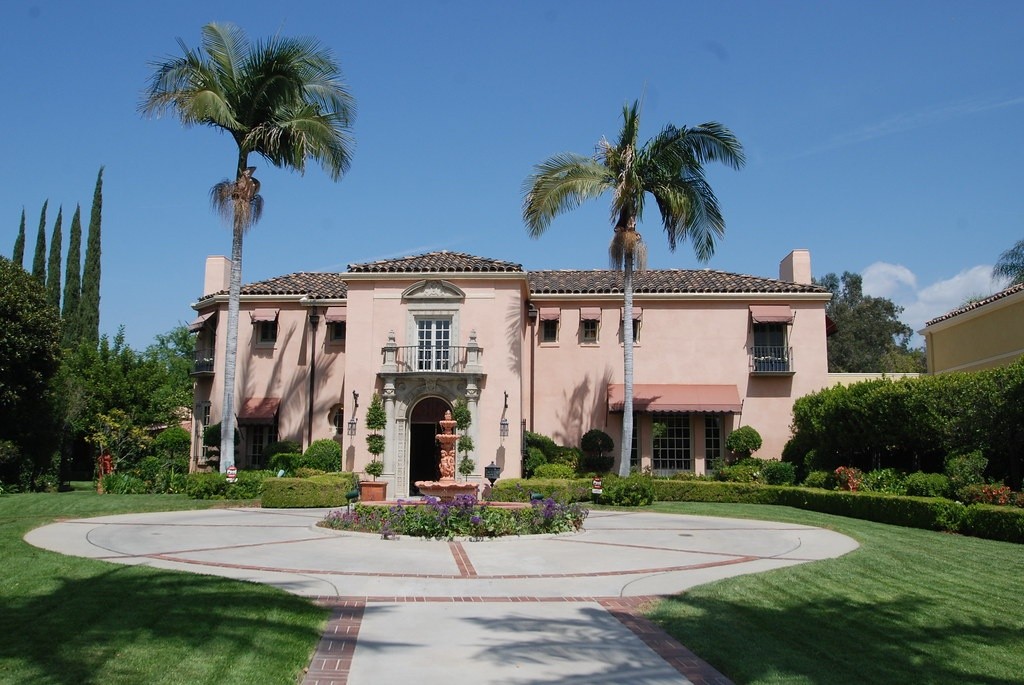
500 417 509 436
347 419 356 435
484 460 501 486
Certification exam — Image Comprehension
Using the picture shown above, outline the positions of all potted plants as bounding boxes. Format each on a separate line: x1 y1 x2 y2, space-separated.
359 391 389 502
452 392 475 505
